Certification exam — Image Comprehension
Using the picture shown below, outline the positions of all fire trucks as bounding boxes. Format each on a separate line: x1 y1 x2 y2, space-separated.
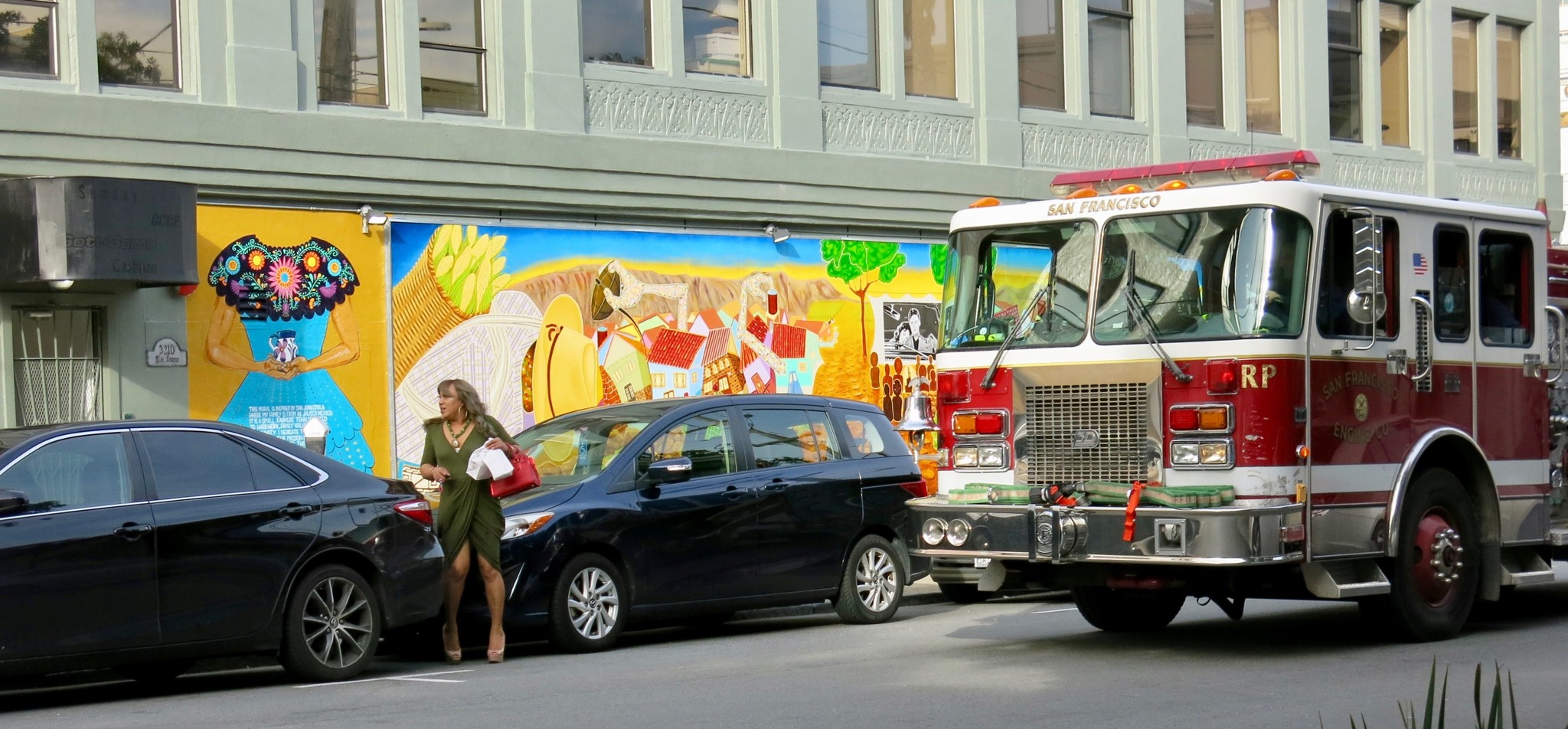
904 150 1567 630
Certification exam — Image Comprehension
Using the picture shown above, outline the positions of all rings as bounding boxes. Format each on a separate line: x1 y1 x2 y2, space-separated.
1266 295 1270 298
439 478 442 483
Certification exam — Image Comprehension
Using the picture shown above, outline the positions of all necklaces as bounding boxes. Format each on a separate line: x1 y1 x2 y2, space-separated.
445 419 471 448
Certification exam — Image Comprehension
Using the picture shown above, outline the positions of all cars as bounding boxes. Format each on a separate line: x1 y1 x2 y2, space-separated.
0 418 450 693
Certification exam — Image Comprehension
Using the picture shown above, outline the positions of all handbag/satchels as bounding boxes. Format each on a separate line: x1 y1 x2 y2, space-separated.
490 443 541 500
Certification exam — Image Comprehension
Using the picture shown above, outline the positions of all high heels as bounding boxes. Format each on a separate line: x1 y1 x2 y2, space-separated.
487 631 505 662
442 625 462 665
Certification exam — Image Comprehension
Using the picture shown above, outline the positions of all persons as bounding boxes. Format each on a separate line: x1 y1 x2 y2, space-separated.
1254 240 1352 335
420 379 523 664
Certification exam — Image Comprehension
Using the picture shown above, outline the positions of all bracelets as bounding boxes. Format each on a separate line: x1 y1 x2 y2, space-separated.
506 445 514 457
430 466 437 482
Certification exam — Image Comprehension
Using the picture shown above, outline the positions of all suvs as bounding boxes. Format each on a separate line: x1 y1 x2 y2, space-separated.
426 392 931 652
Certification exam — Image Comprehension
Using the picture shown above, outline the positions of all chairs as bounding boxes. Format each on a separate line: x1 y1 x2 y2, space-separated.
683 436 724 475
79 457 110 504
750 429 770 466
771 428 804 466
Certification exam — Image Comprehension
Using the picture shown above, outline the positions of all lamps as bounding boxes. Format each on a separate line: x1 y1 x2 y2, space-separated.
764 223 791 244
360 204 388 234
